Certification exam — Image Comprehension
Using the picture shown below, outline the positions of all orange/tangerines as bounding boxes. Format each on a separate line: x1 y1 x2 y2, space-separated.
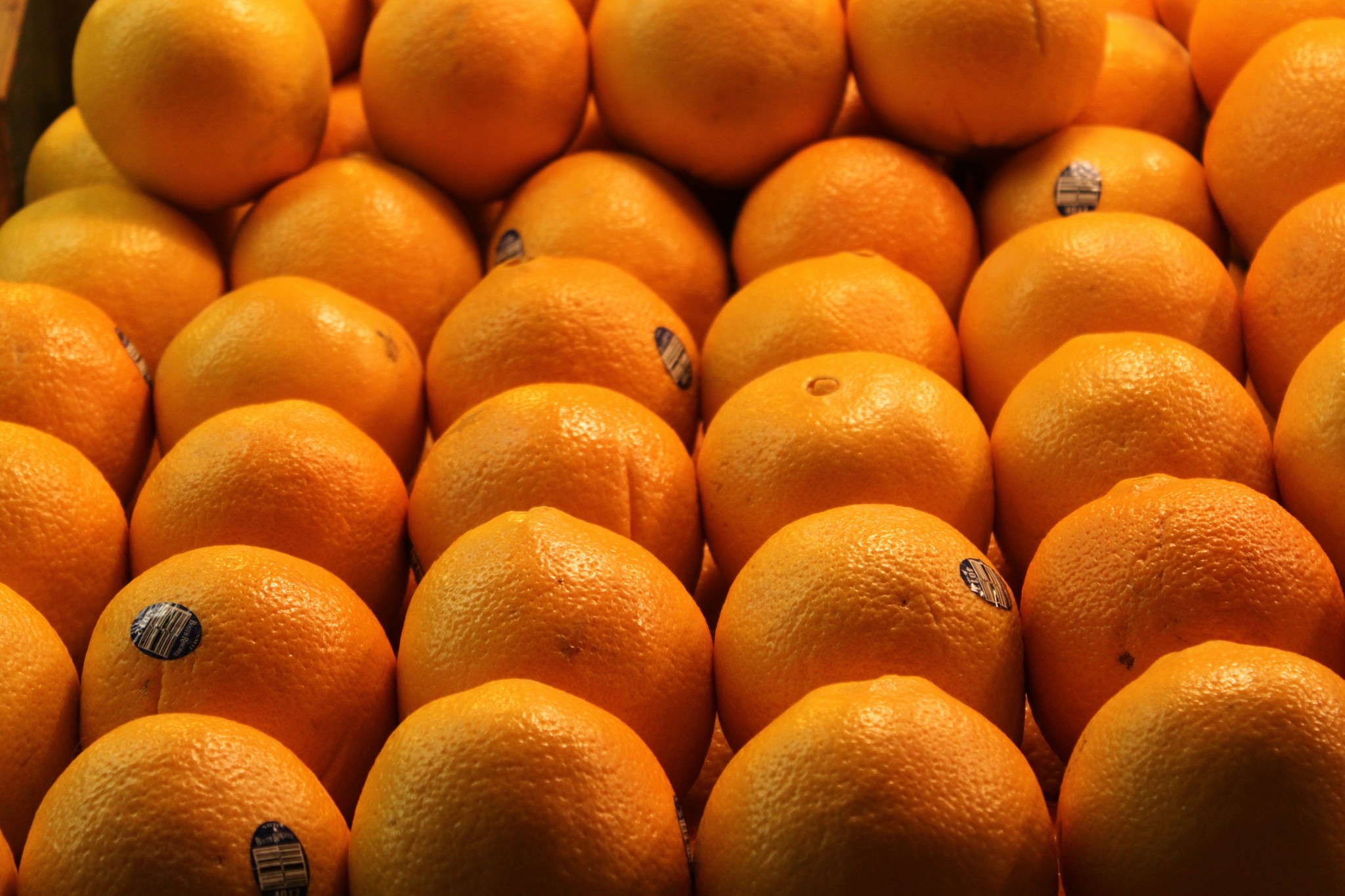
0 0 1345 896
